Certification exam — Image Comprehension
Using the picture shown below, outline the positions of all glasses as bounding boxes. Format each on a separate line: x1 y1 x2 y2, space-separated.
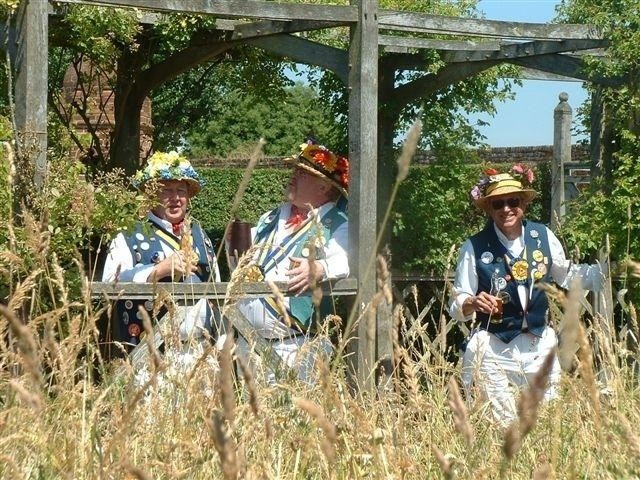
295 167 319 180
487 196 522 211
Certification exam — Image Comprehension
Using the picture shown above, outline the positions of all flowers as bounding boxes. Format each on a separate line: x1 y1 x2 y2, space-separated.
292 136 349 189
131 149 207 187
469 168 496 201
508 163 533 188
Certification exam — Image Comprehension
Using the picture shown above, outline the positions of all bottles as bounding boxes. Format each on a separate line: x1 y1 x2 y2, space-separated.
179 223 193 252
488 273 504 325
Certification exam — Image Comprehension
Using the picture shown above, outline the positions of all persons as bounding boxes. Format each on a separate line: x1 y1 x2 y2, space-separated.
231 141 350 402
101 150 221 427
448 164 640 430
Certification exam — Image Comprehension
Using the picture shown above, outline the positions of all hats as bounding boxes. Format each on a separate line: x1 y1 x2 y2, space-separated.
474 173 535 211
283 144 349 200
134 156 202 199
